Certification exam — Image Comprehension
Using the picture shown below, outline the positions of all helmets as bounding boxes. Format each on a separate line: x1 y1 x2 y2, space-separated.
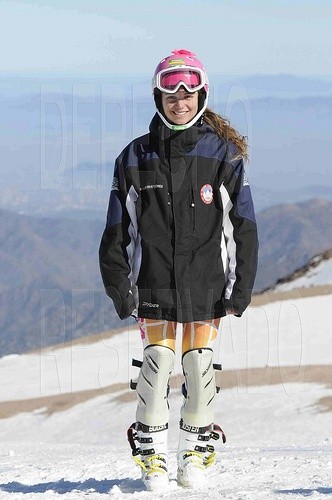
152 48 210 131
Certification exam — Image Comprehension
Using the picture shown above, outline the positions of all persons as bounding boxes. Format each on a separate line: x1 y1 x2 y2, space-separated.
97 50 258 493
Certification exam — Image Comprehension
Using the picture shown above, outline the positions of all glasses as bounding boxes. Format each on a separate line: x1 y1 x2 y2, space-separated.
152 65 209 95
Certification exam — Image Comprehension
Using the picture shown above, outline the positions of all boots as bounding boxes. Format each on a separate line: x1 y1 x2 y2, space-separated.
128 420 170 491
176 418 227 488
